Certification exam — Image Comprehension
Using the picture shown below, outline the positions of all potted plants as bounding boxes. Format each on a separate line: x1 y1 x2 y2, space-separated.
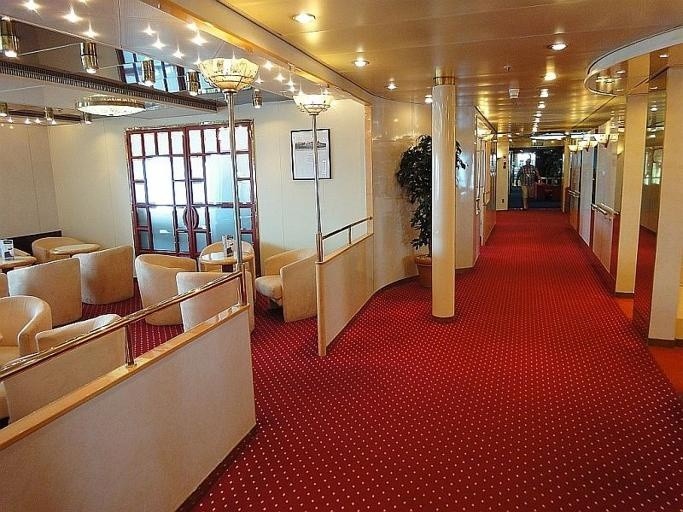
395 135 466 289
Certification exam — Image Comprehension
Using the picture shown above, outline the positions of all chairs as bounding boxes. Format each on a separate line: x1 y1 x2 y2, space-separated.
138 236 321 337
0 234 135 424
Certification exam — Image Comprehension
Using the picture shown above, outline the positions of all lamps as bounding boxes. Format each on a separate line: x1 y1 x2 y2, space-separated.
0 18 199 99
565 133 621 153
292 94 334 235
198 51 257 265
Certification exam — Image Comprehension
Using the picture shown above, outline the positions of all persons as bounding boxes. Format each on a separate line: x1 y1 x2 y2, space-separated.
514 158 540 211
